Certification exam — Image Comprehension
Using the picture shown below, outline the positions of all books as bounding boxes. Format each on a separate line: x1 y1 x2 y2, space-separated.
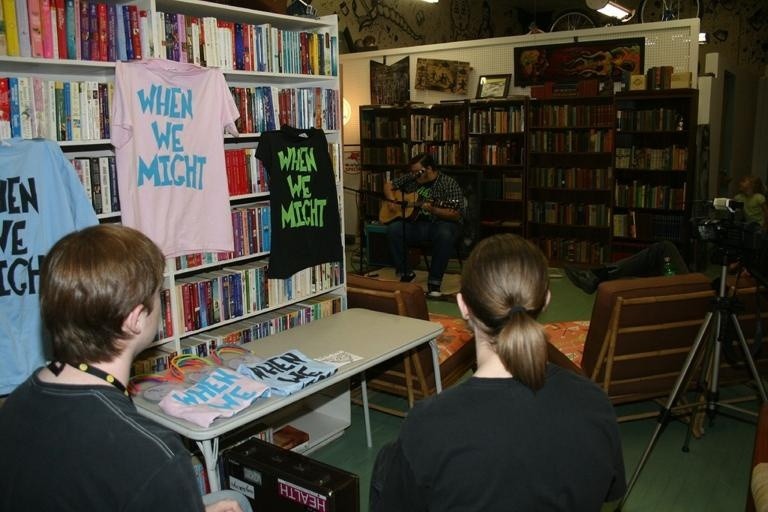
409 115 464 166
469 103 690 265
0 1 336 140
130 147 332 377
67 156 121 215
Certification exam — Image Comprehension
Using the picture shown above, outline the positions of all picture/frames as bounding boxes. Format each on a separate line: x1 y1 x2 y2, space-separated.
514 37 646 86
475 73 512 99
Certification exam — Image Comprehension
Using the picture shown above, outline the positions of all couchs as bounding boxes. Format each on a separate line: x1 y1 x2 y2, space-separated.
535 273 768 438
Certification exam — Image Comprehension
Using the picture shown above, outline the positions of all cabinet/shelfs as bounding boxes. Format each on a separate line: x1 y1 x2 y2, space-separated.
0 0 351 456
359 88 699 269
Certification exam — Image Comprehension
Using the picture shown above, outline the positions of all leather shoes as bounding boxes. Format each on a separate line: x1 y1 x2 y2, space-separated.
563 263 596 294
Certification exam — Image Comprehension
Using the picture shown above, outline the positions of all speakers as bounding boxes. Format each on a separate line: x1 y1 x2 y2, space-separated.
367 223 420 267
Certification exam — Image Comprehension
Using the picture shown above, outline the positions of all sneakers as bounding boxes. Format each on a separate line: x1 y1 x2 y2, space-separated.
425 279 440 298
402 272 418 285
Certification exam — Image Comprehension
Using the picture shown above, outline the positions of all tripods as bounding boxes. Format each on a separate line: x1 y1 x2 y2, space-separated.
616 246 768 512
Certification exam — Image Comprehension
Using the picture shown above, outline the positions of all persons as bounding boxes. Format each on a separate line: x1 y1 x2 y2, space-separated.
383 154 464 297
0 223 254 512
372 232 626 512
565 240 753 295
733 176 768 228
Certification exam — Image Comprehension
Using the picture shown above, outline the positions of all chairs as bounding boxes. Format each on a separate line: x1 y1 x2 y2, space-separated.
344 275 475 418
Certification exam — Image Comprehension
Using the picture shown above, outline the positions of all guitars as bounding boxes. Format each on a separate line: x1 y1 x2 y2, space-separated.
379 182 461 224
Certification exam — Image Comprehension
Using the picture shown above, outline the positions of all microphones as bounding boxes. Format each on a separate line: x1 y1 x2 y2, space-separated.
412 168 426 181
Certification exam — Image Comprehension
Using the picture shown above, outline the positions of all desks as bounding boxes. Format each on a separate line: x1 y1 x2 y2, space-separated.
132 308 445 512
